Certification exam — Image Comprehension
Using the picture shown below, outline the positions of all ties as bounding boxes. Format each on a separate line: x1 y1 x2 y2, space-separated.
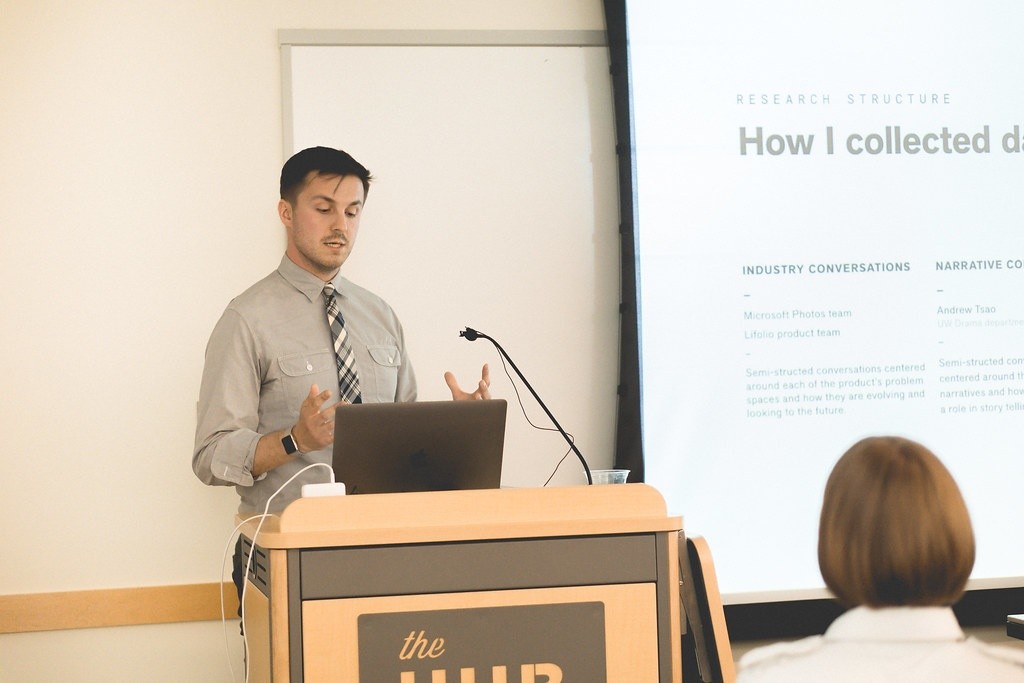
322 284 362 404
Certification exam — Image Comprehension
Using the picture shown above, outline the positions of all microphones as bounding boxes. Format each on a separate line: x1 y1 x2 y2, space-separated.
465 327 478 341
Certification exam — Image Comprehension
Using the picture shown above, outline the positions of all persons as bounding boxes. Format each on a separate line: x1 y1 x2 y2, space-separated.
191 147 490 683
734 435 1024 683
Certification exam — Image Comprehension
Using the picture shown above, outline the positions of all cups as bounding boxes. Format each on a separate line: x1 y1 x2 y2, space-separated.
583 470 630 485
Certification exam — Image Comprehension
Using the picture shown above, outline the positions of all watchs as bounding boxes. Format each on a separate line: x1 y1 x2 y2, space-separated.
280 426 306 458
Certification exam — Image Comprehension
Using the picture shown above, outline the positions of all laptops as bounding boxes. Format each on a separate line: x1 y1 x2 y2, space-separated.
333 399 508 494
